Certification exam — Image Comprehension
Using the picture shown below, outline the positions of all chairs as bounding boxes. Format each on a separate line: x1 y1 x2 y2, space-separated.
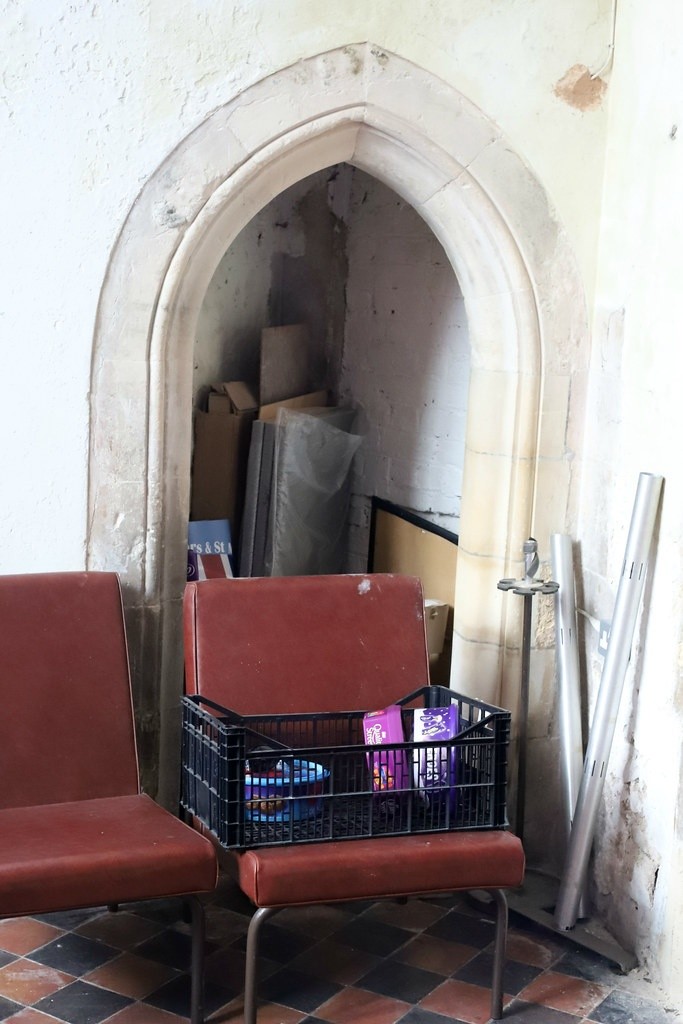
181 572 527 1024
0 570 221 1024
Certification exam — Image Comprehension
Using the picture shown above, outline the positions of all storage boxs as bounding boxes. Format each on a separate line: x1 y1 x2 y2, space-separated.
177 685 512 851
190 379 260 540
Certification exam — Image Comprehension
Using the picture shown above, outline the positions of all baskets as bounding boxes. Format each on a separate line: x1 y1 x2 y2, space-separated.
178 685 512 851
